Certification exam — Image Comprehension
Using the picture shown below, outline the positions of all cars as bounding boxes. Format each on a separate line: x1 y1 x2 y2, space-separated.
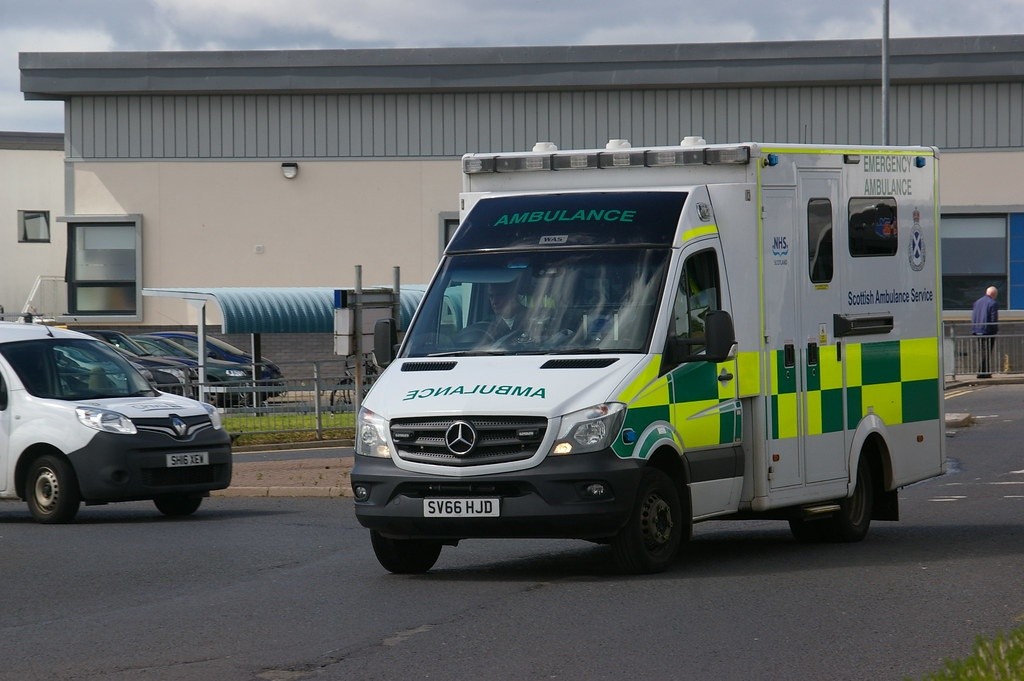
73 329 199 400
1 319 236 524
141 331 285 402
53 344 157 399
129 335 251 408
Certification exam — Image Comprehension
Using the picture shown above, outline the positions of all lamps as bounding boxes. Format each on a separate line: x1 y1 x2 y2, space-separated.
281 162 298 179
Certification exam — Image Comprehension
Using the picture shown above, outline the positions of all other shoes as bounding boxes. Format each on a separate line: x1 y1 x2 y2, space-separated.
977 366 992 379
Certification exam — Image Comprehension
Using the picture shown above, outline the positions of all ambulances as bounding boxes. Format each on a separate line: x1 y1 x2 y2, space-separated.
351 134 946 577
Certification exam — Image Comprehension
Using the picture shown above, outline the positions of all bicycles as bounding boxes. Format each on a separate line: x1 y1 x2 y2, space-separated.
330 352 380 413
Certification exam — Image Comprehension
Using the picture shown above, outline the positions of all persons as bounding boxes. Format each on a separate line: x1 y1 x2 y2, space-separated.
971 285 999 378
484 283 538 353
